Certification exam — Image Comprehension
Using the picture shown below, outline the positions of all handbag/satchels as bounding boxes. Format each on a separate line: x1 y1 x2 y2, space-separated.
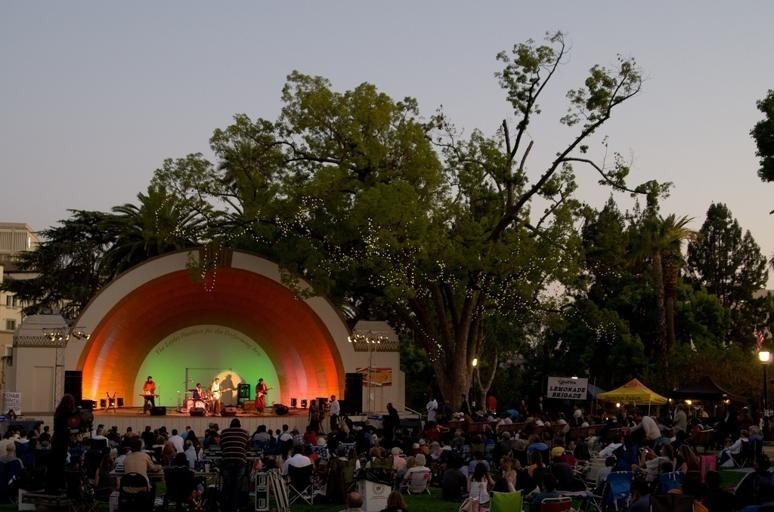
459 497 480 511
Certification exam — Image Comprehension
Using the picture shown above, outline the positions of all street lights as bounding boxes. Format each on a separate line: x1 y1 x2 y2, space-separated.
758 344 770 438
472 358 478 413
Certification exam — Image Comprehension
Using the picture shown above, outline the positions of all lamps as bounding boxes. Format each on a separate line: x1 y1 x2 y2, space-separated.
42 327 90 342
347 329 388 344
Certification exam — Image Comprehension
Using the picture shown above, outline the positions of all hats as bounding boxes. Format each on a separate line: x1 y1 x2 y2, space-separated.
230 419 241 428
415 454 426 464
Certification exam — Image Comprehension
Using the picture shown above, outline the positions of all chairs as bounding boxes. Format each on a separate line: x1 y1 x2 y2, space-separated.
0 422 774 512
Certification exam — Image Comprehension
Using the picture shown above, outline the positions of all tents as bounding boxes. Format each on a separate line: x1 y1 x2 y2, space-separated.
596 378 668 416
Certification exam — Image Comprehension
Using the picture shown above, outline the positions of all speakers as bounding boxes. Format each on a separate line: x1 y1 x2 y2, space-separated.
64 369 82 406
150 406 167 416
237 383 250 405
220 407 237 416
273 404 289 415
344 372 363 416
189 407 206 416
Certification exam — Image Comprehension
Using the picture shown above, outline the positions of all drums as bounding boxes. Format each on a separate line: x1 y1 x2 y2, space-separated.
194 399 205 408
185 399 194 407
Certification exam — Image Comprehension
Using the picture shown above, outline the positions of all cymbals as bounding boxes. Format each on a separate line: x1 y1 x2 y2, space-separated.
188 389 196 391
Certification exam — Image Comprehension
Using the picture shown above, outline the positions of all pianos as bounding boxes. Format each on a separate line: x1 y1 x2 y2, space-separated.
139 394 160 396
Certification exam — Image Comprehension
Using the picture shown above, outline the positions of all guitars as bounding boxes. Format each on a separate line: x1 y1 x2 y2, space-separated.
257 388 273 398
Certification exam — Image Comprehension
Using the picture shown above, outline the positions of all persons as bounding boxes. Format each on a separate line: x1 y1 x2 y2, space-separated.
211 378 221 416
144 376 157 412
0 395 774 512
193 383 203 398
256 378 268 415
